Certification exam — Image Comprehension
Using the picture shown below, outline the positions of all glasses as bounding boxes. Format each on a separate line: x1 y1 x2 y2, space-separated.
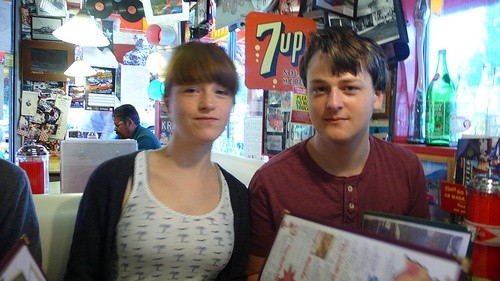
115 120 124 127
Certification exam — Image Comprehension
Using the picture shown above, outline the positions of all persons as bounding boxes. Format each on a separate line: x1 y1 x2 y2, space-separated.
248 25 431 281
63 42 248 281
113 104 162 150
0 158 42 272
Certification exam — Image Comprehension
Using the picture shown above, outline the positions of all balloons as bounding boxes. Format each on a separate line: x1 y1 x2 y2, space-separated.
147 52 165 74
147 24 161 45
148 80 163 100
160 80 165 94
160 52 173 69
159 25 176 46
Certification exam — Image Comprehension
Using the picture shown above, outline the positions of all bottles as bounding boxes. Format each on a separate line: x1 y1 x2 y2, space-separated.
16 139 51 194
425 50 456 146
449 62 500 146
462 174 500 279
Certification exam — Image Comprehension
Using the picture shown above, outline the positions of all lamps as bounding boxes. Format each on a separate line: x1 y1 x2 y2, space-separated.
63 57 97 77
52 0 110 48
